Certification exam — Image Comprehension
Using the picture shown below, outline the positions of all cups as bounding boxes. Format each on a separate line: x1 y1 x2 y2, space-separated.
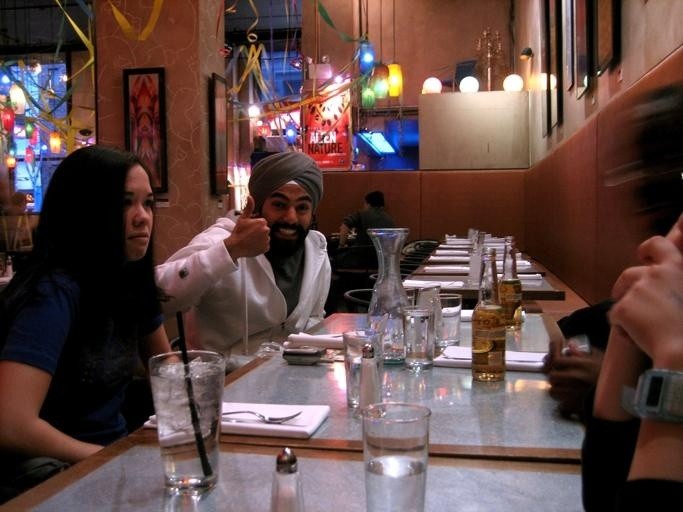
344 279 465 418
148 349 226 495
360 401 432 512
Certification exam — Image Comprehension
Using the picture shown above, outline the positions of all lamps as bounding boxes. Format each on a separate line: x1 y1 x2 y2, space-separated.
423 46 532 93
257 0 403 144
0 0 60 168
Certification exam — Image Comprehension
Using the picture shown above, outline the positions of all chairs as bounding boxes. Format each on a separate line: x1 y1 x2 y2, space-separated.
329 231 565 309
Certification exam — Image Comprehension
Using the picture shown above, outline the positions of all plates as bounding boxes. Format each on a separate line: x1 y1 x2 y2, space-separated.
420 234 543 282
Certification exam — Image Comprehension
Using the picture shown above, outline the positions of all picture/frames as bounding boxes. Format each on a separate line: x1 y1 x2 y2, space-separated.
123 68 168 193
566 0 575 92
593 0 620 77
539 0 562 138
211 71 228 195
574 0 591 100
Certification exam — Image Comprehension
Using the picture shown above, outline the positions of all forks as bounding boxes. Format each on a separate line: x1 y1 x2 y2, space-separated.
221 410 303 424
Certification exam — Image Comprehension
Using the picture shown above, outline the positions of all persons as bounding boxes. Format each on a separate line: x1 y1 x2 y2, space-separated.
544 298 615 415
153 149 331 363
1 144 181 488
583 214 683 511
339 192 391 260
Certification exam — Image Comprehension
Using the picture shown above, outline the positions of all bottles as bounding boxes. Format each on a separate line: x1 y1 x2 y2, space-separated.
6 256 14 272
269 446 303 512
498 236 522 332
366 228 410 365
470 247 506 382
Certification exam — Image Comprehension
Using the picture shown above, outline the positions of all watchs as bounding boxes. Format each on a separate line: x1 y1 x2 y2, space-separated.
618 369 682 421
